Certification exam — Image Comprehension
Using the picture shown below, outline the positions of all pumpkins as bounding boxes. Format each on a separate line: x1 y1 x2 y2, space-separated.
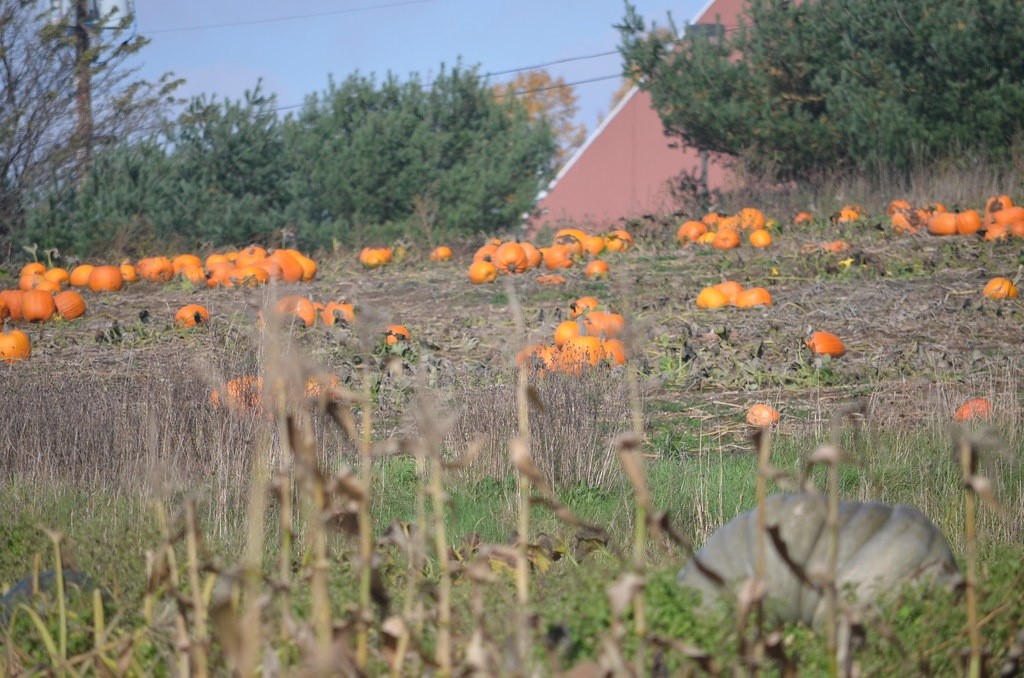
675 196 1024 423
0 226 632 411
